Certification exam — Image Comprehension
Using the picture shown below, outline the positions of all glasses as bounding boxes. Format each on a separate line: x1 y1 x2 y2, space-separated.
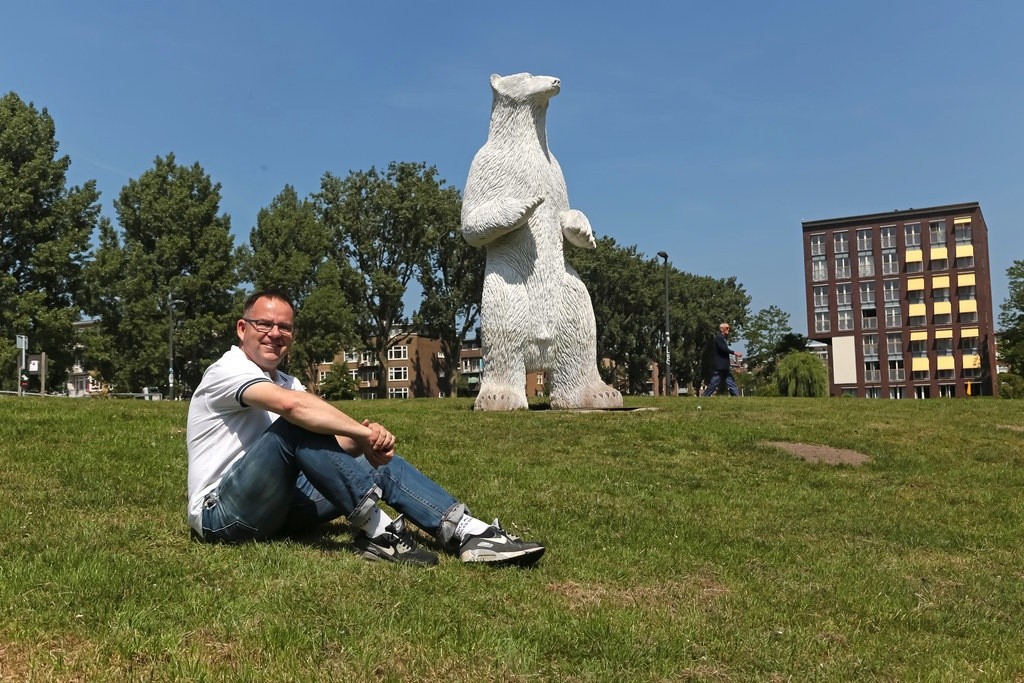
238 317 294 335
722 327 730 330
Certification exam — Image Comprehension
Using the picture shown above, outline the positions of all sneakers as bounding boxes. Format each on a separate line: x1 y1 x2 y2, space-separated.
459 517 545 564
363 514 440 568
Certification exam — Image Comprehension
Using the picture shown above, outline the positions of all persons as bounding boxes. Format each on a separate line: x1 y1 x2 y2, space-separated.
700 322 744 398
184 288 546 572
